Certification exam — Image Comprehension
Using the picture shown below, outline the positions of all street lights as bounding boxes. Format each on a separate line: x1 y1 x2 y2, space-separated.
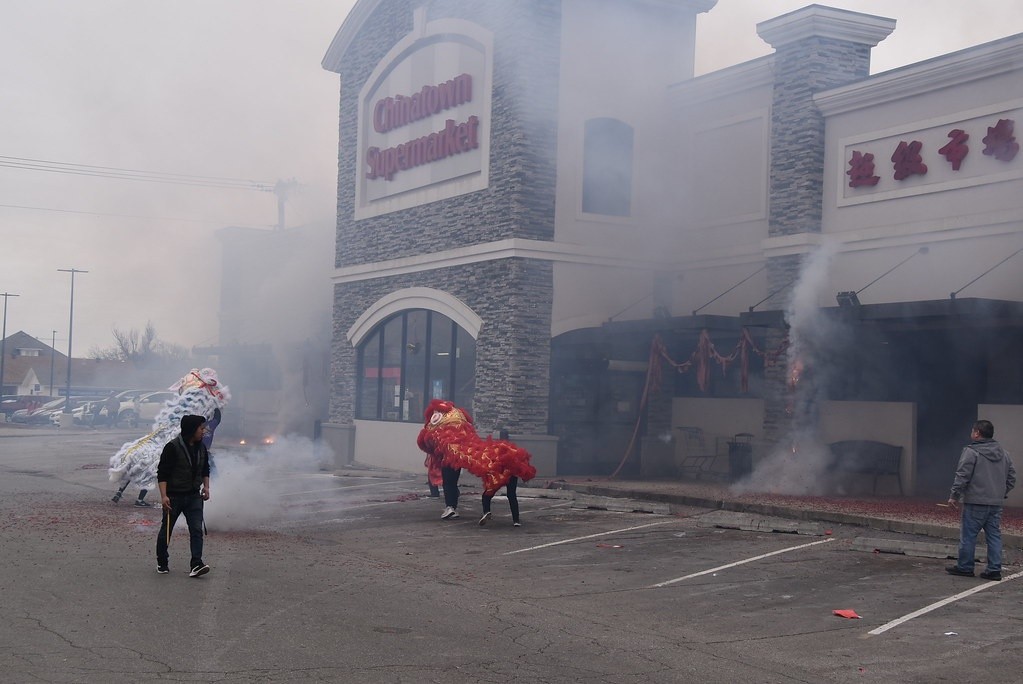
0 293 20 414
58 269 88 414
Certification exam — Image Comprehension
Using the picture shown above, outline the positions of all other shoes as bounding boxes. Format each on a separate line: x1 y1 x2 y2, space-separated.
426 492 440 499
513 520 522 526
478 511 491 525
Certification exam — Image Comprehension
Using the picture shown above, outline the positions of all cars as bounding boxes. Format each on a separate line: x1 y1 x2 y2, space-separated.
41 400 105 426
88 390 153 415
101 392 176 425
12 396 99 423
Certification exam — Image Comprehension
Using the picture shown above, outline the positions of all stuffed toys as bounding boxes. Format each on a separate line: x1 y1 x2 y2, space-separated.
417 399 536 496
107 368 231 489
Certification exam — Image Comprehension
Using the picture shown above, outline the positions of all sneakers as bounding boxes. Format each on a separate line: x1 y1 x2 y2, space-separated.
189 562 209 577
157 563 169 572
441 507 455 518
134 500 151 507
111 496 118 504
451 512 459 518
979 570 1002 580
944 566 975 576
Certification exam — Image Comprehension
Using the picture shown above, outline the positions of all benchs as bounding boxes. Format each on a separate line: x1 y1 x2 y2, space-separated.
826 439 904 500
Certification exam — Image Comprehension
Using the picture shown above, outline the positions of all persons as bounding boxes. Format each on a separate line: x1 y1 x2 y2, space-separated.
156 415 210 579
202 408 222 478
440 465 461 518
105 392 120 428
133 397 140 428
110 480 150 508
478 476 521 527
944 419 1016 581
